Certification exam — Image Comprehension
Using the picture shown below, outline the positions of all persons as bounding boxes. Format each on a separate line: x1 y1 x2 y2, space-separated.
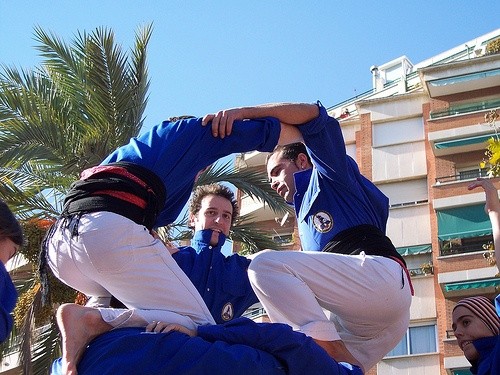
0 194 24 359
35 99 416 374
451 176 500 375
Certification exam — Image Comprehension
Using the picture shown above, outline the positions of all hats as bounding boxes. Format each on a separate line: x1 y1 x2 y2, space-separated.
453 296 500 336
